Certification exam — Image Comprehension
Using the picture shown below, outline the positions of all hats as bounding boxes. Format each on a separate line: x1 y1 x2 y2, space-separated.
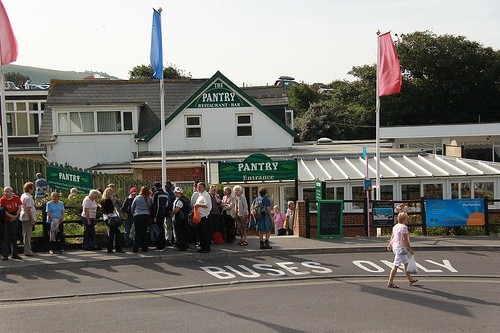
130 188 137 194
173 187 181 192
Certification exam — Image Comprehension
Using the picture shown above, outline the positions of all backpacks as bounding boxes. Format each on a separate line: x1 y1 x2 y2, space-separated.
175 195 191 215
253 197 267 219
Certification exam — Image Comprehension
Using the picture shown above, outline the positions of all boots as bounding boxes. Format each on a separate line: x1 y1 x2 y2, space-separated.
264 239 272 249
260 241 265 249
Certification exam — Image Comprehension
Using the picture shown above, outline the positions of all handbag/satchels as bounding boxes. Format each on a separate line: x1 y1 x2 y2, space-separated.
193 207 201 222
213 231 224 244
108 216 123 227
146 223 160 241
406 252 419 274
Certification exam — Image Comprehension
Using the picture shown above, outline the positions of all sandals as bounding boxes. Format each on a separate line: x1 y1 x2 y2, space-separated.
237 240 248 246
409 279 418 285
388 282 399 288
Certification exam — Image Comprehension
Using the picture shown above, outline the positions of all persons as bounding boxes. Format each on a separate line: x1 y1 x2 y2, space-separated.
164 182 177 247
285 201 295 235
147 183 169 251
220 187 235 243
233 185 249 246
191 181 202 246
101 183 126 253
81 187 103 251
251 188 274 249
386 213 418 288
18 182 37 256
131 186 152 253
120 187 137 248
171 187 188 251
208 185 221 244
34 173 49 202
272 205 287 236
0 187 23 260
194 182 212 253
45 192 65 254
68 188 79 199
394 203 423 213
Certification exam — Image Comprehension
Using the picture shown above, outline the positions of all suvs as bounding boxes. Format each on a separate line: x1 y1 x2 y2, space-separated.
275 76 300 87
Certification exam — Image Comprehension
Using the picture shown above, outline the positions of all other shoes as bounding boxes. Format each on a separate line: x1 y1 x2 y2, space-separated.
48 250 55 254
153 247 165 252
11 255 22 260
2 256 8 259
197 249 210 253
60 249 64 253
25 252 38 257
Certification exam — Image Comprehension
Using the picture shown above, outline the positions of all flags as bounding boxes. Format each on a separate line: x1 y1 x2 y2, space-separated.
377 32 402 97
0 0 19 65
149 7 163 80
